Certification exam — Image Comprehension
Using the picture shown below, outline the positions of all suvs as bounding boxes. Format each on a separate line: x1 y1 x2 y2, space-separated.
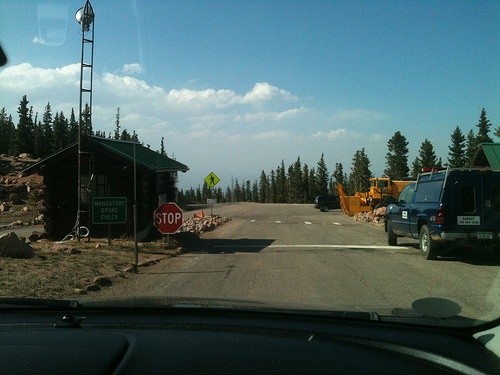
313 195 340 212
384 168 500 266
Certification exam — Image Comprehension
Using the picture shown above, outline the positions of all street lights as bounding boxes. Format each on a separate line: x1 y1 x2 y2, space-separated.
74 1 96 243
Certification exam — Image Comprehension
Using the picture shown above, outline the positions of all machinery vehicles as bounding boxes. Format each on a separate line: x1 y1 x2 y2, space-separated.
337 177 417 218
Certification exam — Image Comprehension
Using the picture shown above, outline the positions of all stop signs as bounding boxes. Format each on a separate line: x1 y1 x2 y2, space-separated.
153 202 184 234
